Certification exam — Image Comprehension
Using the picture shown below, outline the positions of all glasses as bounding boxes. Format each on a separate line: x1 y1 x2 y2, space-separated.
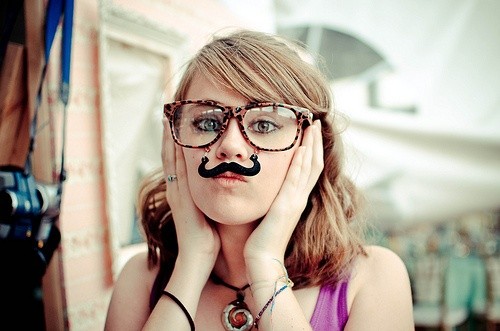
164 100 313 152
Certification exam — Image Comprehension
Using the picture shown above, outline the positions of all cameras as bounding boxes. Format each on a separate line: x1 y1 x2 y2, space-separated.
0 165 60 248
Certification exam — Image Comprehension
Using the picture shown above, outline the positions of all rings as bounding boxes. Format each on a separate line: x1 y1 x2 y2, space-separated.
167 174 177 183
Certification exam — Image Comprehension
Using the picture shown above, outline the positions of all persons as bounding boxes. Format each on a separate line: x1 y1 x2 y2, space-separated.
103 28 417 331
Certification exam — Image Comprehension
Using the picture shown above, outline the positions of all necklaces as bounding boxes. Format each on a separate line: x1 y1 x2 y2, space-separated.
207 273 255 331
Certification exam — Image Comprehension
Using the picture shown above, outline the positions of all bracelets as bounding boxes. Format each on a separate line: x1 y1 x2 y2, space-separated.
245 257 295 328
162 290 196 331
